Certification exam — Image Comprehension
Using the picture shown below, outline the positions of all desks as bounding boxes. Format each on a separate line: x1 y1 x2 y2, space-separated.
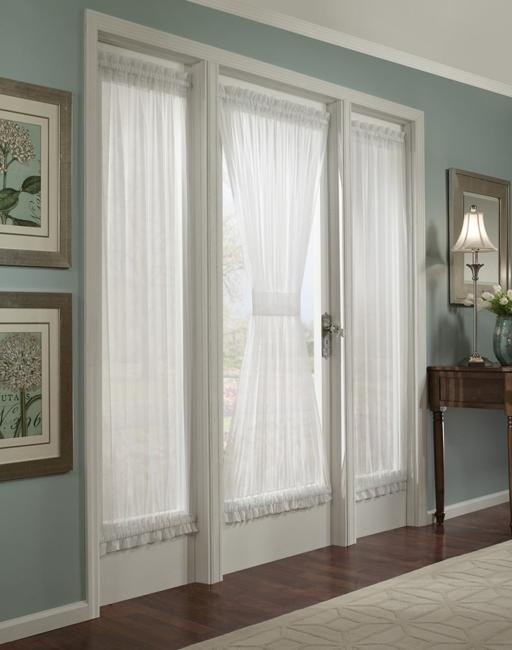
426 365 512 528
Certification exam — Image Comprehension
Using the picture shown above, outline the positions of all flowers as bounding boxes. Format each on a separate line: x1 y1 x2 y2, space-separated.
460 281 511 316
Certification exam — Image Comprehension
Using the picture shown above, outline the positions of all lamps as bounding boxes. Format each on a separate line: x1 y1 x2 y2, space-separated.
452 209 498 364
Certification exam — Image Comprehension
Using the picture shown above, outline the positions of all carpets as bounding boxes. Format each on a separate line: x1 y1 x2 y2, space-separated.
177 540 512 650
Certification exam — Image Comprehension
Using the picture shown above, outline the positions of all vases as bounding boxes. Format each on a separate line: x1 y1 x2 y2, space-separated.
493 314 512 365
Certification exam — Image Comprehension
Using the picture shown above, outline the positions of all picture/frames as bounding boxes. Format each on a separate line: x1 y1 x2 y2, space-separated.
0 76 74 271
448 162 512 310
1 289 76 481
